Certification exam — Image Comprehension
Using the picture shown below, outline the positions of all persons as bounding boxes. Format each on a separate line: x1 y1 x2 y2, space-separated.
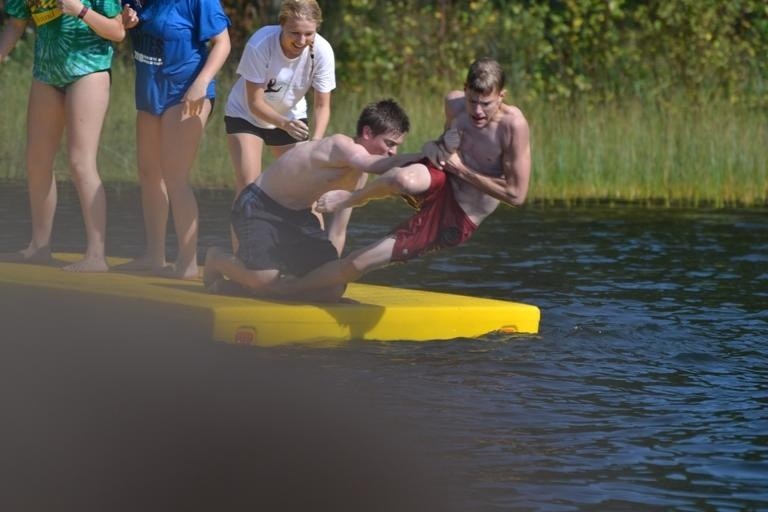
223 0 337 257
0 0 126 272
202 97 411 302
286 57 532 303
111 0 231 279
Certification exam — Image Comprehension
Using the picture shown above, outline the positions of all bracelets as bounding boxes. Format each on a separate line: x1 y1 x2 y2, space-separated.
78 6 88 19
